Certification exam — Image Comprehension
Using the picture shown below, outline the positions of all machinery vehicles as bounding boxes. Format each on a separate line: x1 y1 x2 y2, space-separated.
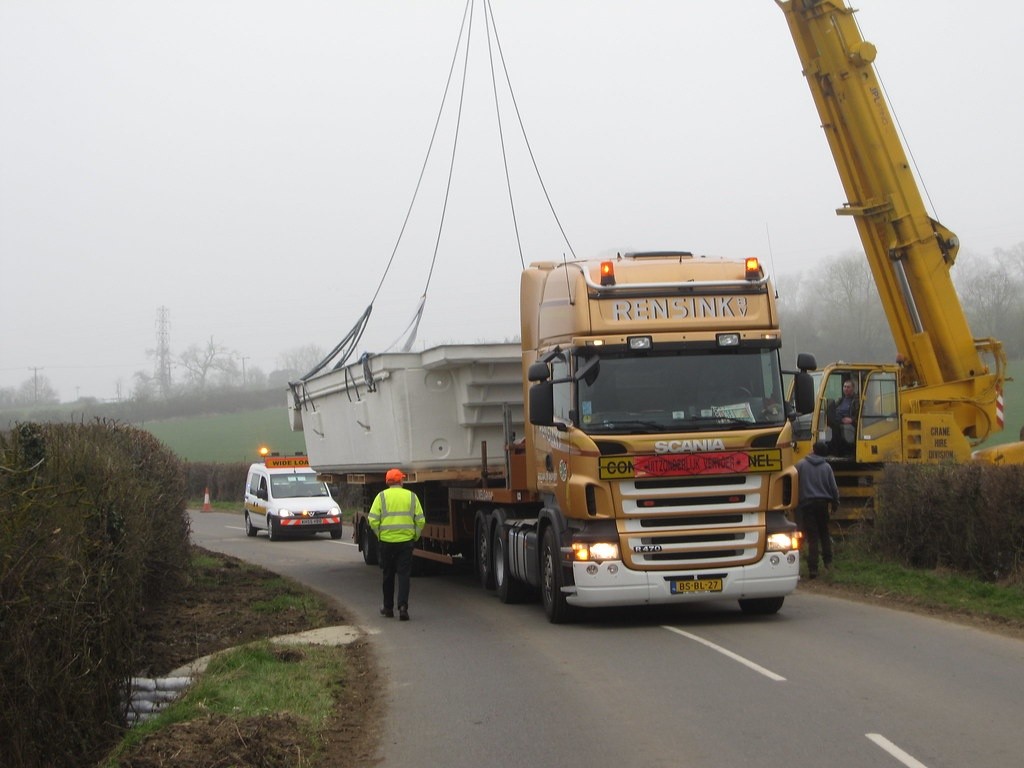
761 0 1023 561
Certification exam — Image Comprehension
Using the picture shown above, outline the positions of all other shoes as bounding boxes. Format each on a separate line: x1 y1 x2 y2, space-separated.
400 608 409 620
809 568 820 578
380 607 394 617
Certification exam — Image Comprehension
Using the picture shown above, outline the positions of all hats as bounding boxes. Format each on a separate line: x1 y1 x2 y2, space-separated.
385 469 406 483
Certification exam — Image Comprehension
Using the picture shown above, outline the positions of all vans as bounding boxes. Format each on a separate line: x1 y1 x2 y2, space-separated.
244 457 342 542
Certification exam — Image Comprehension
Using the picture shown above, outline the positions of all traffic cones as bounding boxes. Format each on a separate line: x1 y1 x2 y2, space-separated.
200 487 213 512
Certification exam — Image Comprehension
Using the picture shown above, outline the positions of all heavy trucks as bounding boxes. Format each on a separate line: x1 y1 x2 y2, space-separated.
352 251 818 629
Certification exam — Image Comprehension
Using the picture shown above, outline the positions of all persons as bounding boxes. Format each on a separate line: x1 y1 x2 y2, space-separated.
795 441 838 579
368 469 425 621
835 379 859 442
